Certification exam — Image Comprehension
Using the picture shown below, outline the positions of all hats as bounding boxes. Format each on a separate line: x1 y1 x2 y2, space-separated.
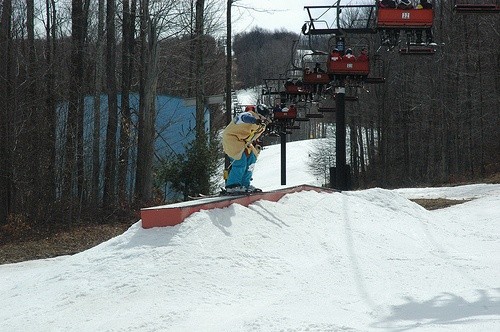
257 103 269 116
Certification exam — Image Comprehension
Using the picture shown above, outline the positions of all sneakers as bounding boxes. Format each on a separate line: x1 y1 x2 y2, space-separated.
226 185 261 196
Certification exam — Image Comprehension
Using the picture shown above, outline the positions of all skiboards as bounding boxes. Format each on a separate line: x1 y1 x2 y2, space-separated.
187 191 275 200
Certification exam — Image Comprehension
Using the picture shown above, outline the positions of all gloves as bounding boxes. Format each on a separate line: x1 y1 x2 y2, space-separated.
256 119 265 126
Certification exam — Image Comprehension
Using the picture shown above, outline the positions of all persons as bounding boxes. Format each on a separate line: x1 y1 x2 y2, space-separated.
326 0 437 76
222 61 324 198
